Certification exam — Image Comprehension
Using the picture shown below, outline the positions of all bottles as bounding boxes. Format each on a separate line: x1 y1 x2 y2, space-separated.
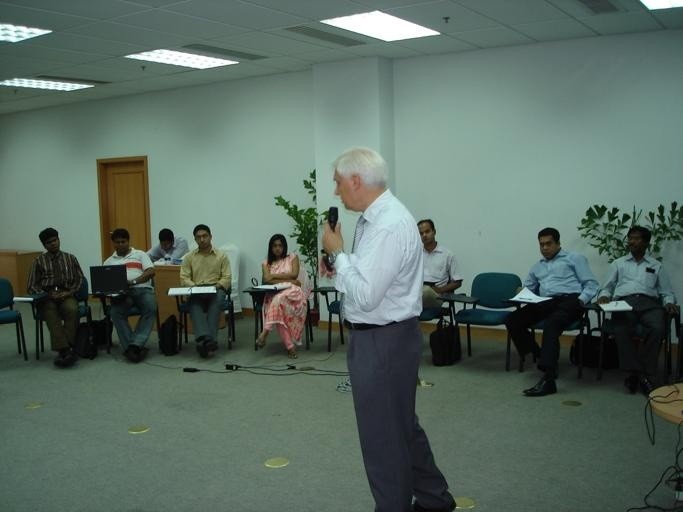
164 254 171 265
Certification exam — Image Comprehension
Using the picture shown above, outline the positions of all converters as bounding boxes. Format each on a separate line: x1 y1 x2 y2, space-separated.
183 368 196 372
225 364 237 370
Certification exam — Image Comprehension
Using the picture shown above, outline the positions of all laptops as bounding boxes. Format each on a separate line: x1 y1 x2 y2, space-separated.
90 265 129 291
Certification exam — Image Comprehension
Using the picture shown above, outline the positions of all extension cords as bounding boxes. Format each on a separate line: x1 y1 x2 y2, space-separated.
675 469 683 501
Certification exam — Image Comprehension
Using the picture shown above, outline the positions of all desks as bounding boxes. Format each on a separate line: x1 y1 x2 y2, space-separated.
648 383 682 505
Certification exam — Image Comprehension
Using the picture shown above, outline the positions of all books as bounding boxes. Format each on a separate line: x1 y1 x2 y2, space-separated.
598 298 632 312
251 281 291 292
508 286 552 305
167 286 216 298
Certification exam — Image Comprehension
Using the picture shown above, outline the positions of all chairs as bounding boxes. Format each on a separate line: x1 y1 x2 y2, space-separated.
417 270 455 331
592 295 678 388
93 270 163 355
31 272 94 360
174 285 237 351
523 287 591 382
242 277 313 347
318 285 345 352
2 277 30 362
455 271 523 373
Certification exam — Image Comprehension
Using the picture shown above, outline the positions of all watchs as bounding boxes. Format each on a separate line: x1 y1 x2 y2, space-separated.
131 279 137 286
327 248 345 266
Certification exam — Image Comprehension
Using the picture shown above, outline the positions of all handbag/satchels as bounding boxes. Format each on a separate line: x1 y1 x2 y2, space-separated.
75 326 99 360
430 319 460 365
570 328 612 368
161 315 177 356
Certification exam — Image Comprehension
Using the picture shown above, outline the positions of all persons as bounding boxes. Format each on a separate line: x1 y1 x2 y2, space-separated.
178 223 230 358
101 227 156 363
594 224 680 398
317 145 457 512
254 233 308 359
415 218 463 310
26 227 85 368
503 226 601 397
144 227 188 265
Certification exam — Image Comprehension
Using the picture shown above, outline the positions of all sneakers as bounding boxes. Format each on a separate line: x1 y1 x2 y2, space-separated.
197 337 217 357
123 344 148 362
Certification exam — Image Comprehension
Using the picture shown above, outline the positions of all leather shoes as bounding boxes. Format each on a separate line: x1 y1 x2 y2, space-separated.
625 373 657 394
524 378 556 395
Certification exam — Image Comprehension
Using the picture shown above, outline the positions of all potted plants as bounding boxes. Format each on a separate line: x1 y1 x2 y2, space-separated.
271 167 318 327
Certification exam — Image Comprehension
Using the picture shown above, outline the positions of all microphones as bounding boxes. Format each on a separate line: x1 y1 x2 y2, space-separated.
328 207 338 272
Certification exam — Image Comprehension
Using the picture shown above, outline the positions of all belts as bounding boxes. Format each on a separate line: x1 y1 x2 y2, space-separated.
344 318 396 330
